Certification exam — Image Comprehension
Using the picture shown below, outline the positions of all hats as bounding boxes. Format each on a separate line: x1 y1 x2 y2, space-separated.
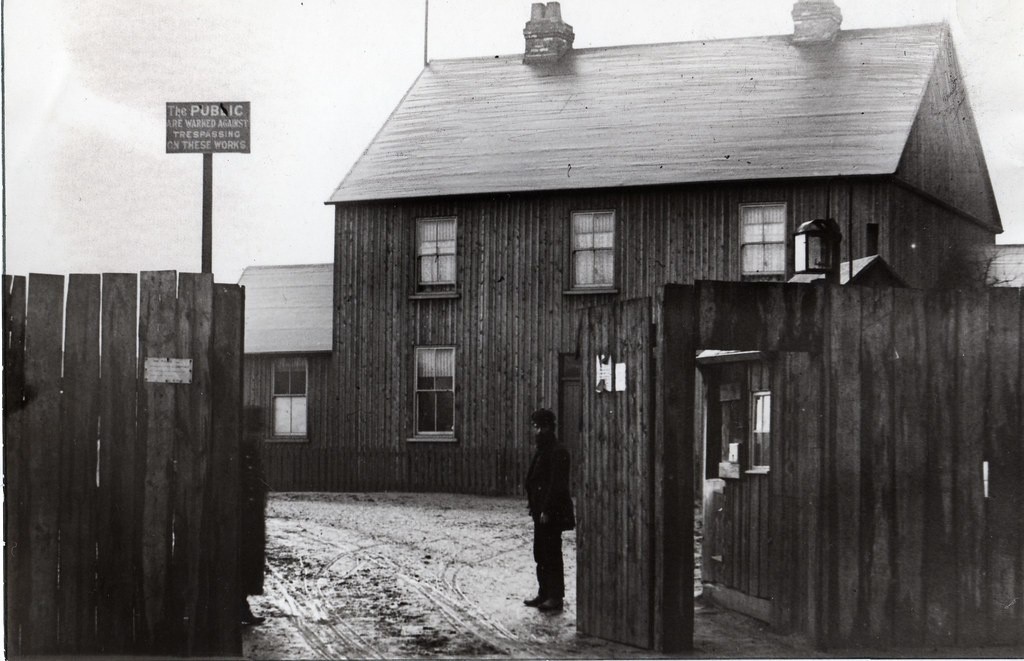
531 408 556 422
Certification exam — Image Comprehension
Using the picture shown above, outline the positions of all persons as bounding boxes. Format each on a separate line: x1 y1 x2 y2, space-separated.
522 408 576 612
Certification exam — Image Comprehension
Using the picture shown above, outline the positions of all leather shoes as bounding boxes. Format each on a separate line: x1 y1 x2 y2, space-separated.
523 595 563 611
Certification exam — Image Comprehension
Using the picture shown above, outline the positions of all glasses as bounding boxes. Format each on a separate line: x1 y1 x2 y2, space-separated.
533 424 552 431
